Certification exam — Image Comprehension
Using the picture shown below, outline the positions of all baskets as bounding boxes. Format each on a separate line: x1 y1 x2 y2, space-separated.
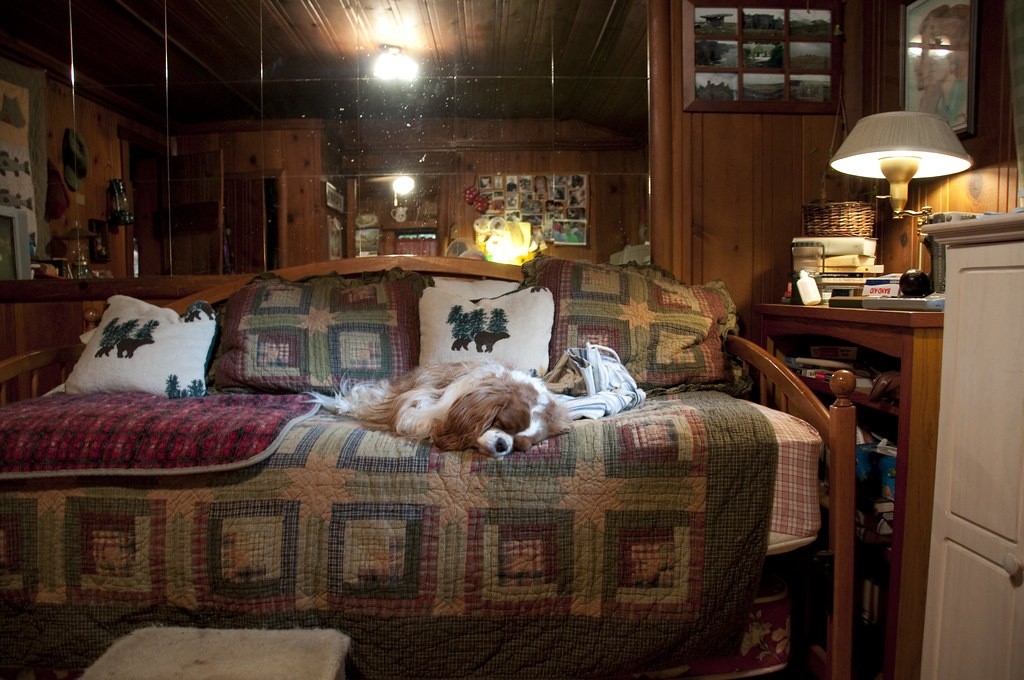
804 158 875 238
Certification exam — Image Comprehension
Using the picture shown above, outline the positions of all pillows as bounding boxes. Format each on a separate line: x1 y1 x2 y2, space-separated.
42 295 217 400
431 276 519 300
418 285 556 379
205 267 435 397
520 250 754 398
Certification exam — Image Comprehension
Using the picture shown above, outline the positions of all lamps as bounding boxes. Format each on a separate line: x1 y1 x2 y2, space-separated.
829 111 975 299
107 177 137 226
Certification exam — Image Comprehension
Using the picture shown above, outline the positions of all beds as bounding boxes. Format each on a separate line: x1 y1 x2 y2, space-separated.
0 252 857 680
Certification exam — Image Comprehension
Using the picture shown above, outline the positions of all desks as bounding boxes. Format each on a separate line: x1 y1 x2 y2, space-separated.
753 302 944 680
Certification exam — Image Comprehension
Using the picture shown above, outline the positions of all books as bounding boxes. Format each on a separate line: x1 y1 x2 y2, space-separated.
791 236 902 304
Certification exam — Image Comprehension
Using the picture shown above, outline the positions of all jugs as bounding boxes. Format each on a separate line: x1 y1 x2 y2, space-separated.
391 207 408 222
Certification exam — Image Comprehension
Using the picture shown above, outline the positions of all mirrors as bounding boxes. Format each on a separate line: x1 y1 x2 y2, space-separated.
0 0 650 301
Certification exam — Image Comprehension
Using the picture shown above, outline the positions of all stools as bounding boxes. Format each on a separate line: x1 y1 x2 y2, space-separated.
78 620 351 680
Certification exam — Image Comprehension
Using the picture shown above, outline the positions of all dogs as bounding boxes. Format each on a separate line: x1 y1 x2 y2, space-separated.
302 362 572 457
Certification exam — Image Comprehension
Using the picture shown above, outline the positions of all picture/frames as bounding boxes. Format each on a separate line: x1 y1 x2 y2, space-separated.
324 179 346 216
88 218 112 262
899 0 977 141
476 171 590 243
553 219 590 245
681 0 844 115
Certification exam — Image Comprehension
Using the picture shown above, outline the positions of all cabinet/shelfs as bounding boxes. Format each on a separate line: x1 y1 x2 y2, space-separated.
920 212 1024 680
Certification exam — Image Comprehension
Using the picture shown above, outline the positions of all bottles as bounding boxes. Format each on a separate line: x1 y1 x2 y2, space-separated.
133 237 138 277
797 270 821 306
